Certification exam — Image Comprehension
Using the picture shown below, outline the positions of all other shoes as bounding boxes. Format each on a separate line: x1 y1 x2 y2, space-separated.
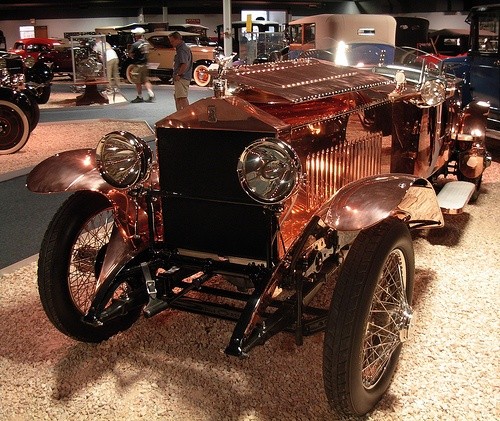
101 89 114 94
114 88 120 93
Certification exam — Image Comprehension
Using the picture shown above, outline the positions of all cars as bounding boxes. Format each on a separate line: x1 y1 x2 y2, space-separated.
0 47 53 155
23 32 489 418
415 29 495 68
0 13 434 67
8 38 61 65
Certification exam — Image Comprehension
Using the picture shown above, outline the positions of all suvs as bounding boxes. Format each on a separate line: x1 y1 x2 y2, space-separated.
125 32 221 87
464 0 500 140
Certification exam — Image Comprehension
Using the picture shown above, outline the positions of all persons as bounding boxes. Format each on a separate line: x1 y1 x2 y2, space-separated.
128 28 155 103
169 32 193 112
94 38 121 95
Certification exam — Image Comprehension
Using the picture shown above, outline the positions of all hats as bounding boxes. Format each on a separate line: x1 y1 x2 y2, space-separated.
131 28 145 34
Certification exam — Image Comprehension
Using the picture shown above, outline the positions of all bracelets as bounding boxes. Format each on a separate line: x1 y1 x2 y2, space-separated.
176 73 180 77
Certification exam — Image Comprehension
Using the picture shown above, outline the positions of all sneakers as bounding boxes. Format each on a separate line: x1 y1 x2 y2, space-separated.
130 95 143 103
144 96 155 102
